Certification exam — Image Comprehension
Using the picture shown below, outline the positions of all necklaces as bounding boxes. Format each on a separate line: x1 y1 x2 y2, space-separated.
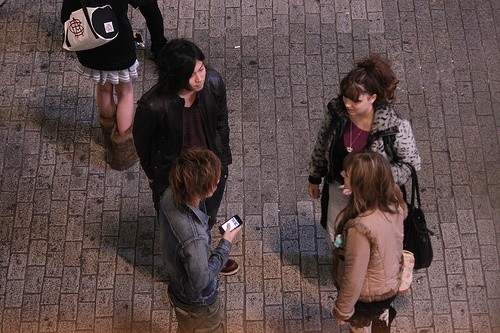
346 119 365 152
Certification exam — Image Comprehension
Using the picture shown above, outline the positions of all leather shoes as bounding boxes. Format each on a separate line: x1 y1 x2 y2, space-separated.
219 259 239 276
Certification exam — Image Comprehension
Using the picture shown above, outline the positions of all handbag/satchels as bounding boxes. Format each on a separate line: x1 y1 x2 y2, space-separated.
60 0 120 53
398 249 416 293
393 161 434 270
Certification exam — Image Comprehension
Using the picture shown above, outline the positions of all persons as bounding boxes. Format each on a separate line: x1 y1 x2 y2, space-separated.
307 53 423 275
60 0 169 171
332 151 409 333
159 146 244 333
131 38 240 276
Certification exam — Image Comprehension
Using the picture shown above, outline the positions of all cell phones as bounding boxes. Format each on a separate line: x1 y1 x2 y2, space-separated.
219 215 242 235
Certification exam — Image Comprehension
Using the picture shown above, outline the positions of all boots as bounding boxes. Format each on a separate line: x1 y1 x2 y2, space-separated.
96 104 140 171
